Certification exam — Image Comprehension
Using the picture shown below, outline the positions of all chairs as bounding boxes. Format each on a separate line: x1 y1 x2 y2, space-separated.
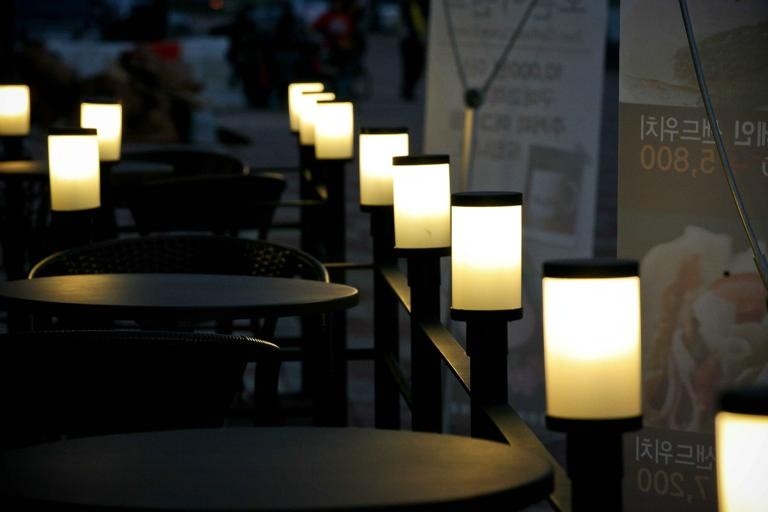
0 146 329 454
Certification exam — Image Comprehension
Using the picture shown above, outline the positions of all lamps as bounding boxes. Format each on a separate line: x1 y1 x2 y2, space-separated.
539 257 646 432
712 407 768 512
355 127 410 211
286 81 355 163
448 191 527 323
391 152 451 256
0 78 124 217
